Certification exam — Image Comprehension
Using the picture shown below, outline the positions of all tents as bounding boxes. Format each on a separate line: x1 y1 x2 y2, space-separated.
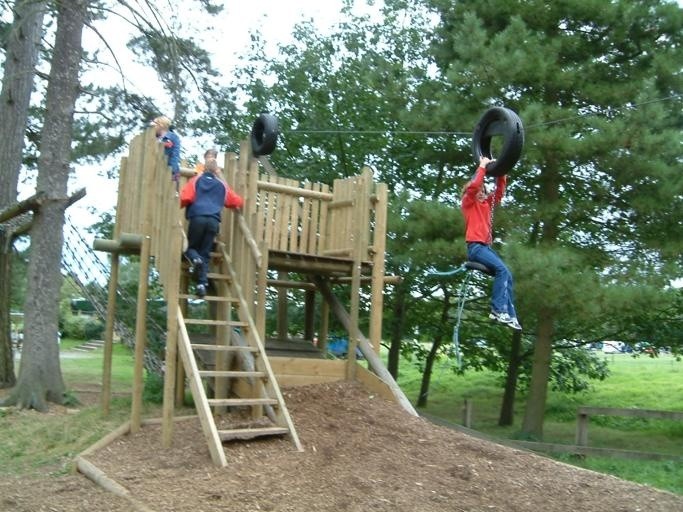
328 337 365 359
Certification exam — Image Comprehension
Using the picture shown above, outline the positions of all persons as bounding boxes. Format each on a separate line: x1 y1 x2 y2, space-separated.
10 319 24 343
180 156 245 297
154 115 181 195
459 155 524 332
57 330 62 345
192 148 228 181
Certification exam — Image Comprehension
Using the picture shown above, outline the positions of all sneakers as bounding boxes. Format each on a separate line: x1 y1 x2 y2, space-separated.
188 258 207 296
488 309 522 332
